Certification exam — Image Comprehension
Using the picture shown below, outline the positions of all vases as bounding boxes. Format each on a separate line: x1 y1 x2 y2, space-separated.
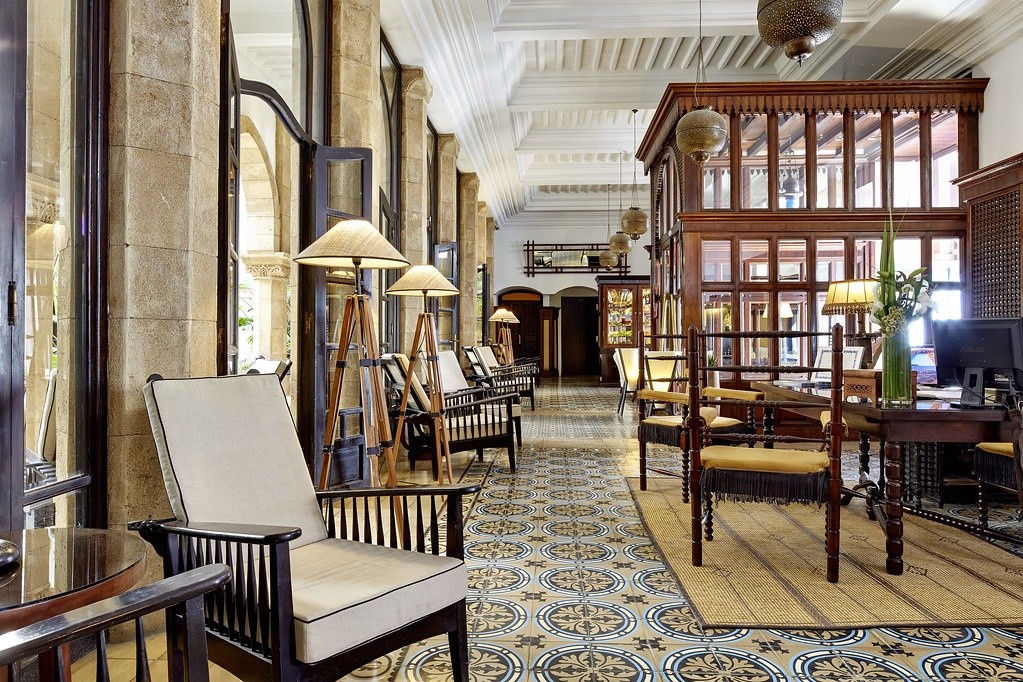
881 323 913 405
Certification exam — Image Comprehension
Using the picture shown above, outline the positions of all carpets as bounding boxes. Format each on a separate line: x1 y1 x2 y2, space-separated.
625 476 1023 629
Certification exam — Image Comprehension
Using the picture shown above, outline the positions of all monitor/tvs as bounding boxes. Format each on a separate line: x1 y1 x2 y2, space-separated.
930 317 1023 411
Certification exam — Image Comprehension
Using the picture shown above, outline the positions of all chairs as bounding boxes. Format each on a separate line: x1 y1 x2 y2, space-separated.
637 330 766 505
687 324 846 583
973 443 1023 528
417 350 523 461
380 352 519 481
462 345 540 411
124 369 483 682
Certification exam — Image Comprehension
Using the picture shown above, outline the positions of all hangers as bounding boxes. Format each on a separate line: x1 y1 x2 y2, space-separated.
783 136 799 176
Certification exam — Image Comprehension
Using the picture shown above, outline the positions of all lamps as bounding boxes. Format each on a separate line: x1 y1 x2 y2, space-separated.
762 303 794 319
609 152 633 259
599 183 618 272
385 265 461 501
291 215 413 550
757 0 844 68
612 346 683 417
675 0 728 166
820 279 888 368
488 308 516 372
620 109 650 243
499 310 521 370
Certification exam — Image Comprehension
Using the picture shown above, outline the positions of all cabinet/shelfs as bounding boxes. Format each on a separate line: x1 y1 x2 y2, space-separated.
594 275 651 387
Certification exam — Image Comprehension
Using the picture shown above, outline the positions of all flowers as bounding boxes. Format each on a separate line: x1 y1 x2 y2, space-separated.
865 198 939 400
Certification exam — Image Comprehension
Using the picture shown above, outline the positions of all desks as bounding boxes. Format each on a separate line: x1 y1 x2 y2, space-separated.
0 528 147 682
750 380 1023 575
